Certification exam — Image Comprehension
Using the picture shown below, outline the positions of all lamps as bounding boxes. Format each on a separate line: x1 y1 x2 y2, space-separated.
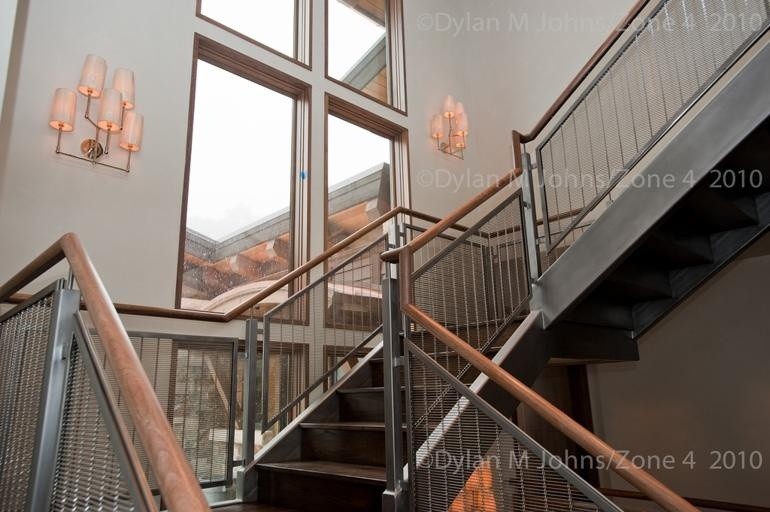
430 94 469 160
48 52 144 174
